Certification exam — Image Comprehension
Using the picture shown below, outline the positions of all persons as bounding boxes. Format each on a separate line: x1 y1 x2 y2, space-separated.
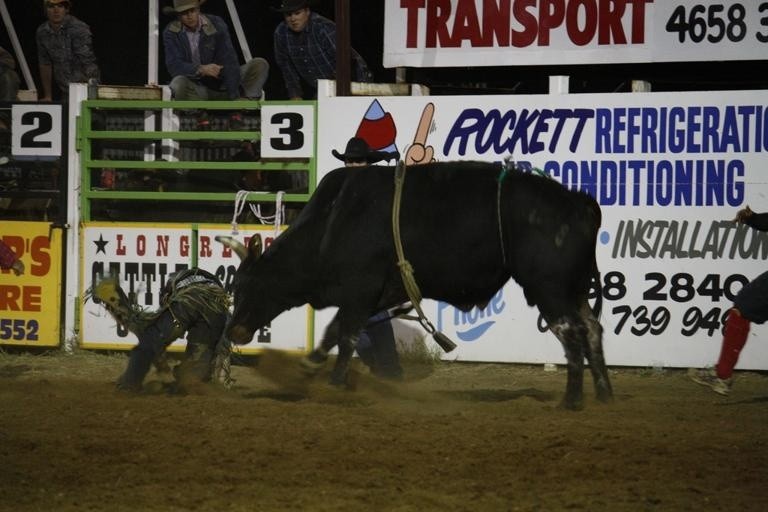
116 267 231 394
685 205 768 396
35 0 115 190
299 138 406 381
0 47 22 132
275 0 374 101
163 0 270 131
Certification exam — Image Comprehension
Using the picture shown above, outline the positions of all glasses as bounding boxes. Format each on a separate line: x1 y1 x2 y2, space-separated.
42 0 66 10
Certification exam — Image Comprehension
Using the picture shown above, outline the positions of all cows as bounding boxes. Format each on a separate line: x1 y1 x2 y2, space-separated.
214 159 615 414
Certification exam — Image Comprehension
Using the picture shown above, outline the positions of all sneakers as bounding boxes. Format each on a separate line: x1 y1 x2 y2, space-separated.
100 168 114 188
229 112 242 129
197 113 211 130
302 349 328 369
686 362 736 398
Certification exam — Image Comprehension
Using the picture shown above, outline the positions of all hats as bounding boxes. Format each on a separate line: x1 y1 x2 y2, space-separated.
334 138 383 163
272 0 312 15
161 0 206 14
